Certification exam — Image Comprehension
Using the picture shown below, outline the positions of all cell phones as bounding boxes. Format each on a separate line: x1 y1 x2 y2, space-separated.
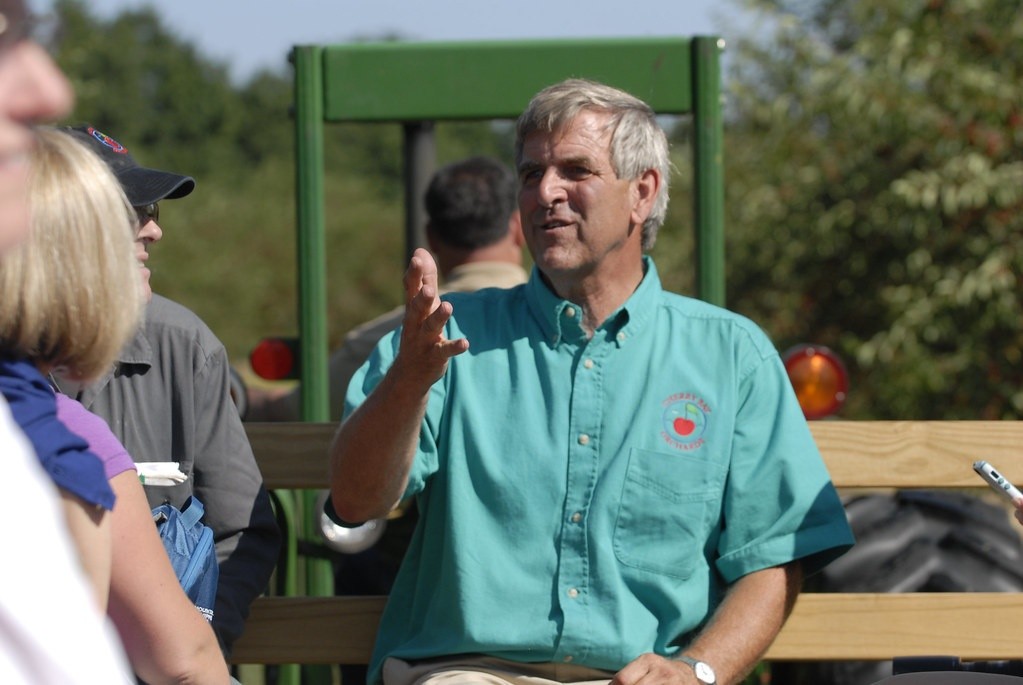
973 461 1023 502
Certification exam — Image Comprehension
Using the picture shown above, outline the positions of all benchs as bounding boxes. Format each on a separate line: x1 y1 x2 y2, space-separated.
226 419 1023 661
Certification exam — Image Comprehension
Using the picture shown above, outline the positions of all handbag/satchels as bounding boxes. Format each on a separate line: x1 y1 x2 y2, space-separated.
150 494 220 624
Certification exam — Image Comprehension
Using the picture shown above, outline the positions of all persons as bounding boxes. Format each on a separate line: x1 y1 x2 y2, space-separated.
0 0 284 685
326 80 856 685
281 156 530 422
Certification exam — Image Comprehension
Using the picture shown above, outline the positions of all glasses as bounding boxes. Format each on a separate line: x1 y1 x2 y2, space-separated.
134 202 159 228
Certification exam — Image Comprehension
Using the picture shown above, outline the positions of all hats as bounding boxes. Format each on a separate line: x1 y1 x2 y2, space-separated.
57 125 196 206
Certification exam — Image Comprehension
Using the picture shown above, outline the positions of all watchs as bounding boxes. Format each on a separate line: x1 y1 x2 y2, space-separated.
674 655 717 685
